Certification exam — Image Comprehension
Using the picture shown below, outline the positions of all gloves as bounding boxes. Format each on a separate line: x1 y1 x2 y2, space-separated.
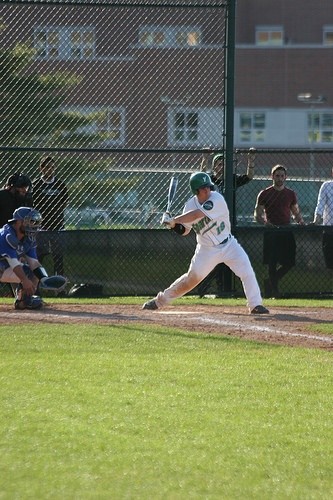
160 212 170 227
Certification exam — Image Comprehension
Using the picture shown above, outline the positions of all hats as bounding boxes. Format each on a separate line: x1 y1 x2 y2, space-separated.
212 154 223 170
8 207 34 222
8 173 28 187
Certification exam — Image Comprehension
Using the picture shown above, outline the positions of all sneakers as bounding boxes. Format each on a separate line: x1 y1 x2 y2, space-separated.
142 300 158 310
250 305 270 314
14 297 41 309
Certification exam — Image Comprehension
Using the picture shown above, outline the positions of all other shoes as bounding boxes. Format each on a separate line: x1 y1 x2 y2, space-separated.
271 287 281 298
265 289 271 298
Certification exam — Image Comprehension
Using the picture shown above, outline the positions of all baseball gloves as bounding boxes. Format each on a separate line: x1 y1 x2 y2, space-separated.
40 276 69 288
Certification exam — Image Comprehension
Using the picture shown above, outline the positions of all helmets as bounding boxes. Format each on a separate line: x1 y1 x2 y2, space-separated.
190 173 214 195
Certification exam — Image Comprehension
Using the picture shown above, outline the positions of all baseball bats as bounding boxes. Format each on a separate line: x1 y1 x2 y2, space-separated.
161 176 177 227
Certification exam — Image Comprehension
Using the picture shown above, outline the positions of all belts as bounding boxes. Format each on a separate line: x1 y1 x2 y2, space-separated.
220 234 231 245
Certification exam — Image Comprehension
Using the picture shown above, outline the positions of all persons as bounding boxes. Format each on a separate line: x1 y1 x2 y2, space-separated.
253 165 308 298
0 157 67 309
141 171 269 314
310 180 333 270
199 145 257 295
